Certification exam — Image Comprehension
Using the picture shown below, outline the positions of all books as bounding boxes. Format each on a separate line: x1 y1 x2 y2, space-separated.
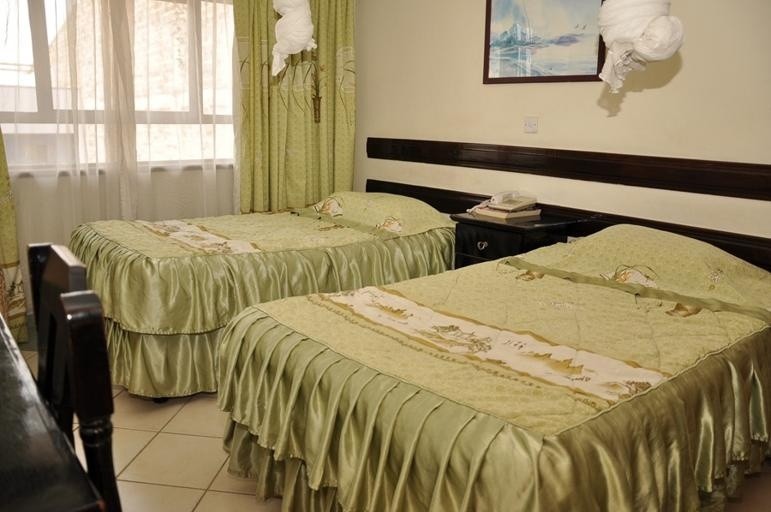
473 213 542 223
476 206 543 219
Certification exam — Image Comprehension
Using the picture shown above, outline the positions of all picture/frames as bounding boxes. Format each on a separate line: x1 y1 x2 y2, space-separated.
483 1 606 85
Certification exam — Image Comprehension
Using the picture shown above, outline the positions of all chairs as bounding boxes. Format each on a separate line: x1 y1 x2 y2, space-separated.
25 243 123 511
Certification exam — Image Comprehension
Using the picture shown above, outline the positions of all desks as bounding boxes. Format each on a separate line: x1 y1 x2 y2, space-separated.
1 298 105 511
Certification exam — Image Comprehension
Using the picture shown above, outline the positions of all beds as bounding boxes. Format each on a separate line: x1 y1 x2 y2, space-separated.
226 236 771 512
74 206 460 404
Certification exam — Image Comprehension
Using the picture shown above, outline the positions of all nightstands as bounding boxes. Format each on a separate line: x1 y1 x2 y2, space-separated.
450 212 583 270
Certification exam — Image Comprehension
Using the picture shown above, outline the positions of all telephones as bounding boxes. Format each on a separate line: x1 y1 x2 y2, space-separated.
488 190 536 211
